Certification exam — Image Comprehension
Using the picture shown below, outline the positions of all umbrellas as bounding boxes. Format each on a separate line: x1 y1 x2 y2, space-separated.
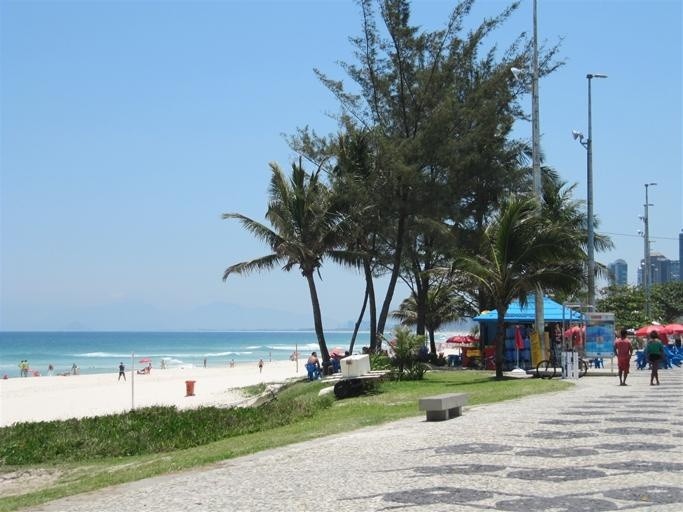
636 325 673 336
664 323 682 336
459 336 481 346
514 324 525 368
445 336 464 356
138 358 152 363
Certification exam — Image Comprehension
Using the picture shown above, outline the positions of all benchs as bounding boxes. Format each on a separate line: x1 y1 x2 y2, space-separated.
417 392 469 422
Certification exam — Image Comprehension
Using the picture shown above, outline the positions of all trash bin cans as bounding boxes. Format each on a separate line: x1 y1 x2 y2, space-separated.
448 355 459 368
184 381 196 396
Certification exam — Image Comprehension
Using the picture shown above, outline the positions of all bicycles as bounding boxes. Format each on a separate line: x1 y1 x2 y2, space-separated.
535 345 588 381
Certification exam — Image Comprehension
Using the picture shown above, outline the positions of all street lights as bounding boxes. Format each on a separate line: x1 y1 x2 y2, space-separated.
571 73 606 371
633 180 658 324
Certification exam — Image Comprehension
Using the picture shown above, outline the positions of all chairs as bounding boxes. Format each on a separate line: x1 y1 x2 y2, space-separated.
304 363 321 381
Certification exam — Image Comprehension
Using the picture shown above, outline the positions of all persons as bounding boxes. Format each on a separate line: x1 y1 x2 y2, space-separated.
329 351 350 360
613 329 633 386
563 322 583 352
118 362 126 382
550 322 561 363
46 364 54 376
257 359 264 373
675 335 682 349
22 360 29 377
580 322 586 337
136 366 149 374
18 360 24 377
307 351 322 378
646 330 665 386
140 362 152 371
71 363 77 375
